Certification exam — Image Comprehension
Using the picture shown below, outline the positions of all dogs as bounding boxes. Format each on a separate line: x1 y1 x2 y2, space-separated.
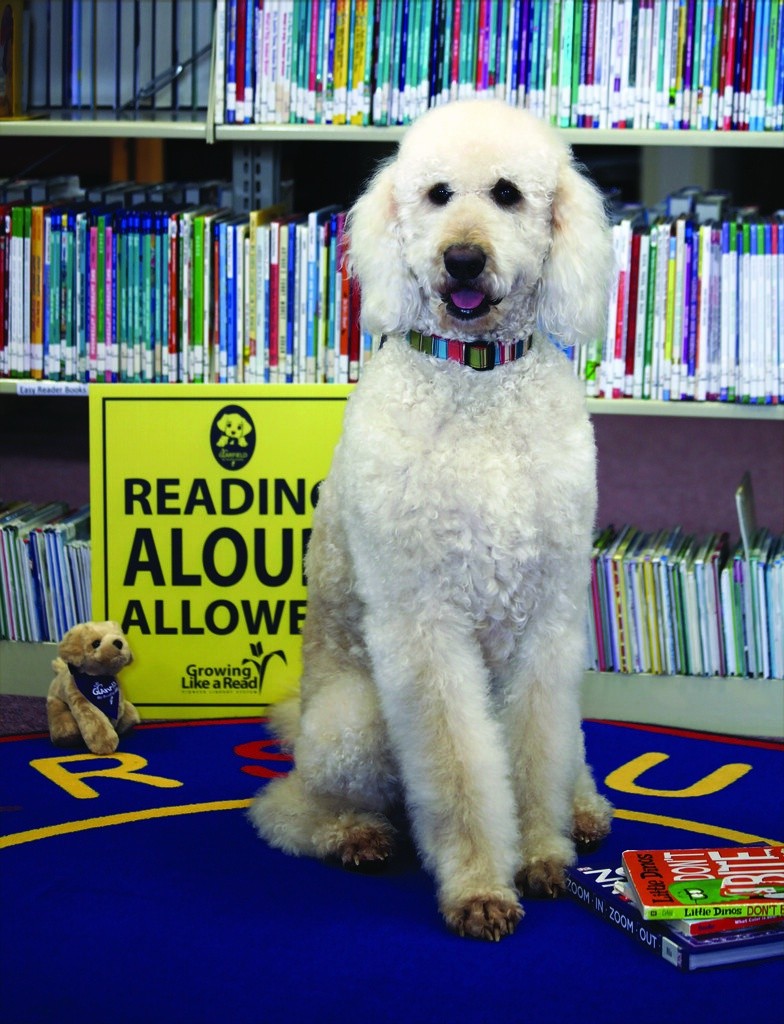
244 101 616 941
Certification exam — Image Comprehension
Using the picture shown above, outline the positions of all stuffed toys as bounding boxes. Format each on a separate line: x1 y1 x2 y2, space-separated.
48 621 140 754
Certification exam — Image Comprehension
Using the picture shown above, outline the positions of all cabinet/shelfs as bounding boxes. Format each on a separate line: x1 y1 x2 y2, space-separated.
0 0 784 742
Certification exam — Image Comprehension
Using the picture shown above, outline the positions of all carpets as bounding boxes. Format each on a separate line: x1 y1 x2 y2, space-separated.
0 716 784 1024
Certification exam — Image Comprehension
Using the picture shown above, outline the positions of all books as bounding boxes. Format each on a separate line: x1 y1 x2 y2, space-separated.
0 175 388 385
539 186 784 406
586 470 784 680
0 500 92 644
557 846 784 973
0 0 784 132
